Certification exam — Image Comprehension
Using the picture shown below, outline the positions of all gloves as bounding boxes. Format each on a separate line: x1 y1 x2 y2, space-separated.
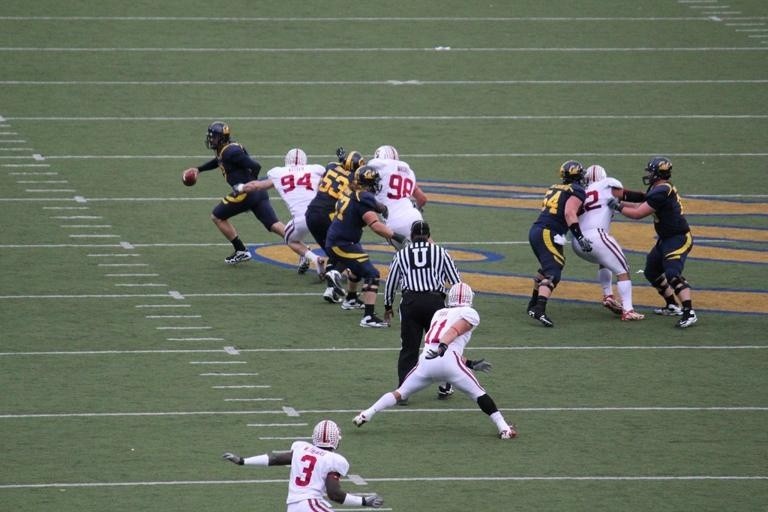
579 236 594 252
233 184 245 195
606 196 625 212
465 358 492 373
425 343 447 360
554 234 571 245
336 147 344 162
382 206 388 219
362 496 384 508
222 453 243 466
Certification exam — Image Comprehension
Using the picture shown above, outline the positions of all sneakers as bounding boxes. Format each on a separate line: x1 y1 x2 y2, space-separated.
621 309 645 321
603 295 623 314
500 428 516 440
353 412 368 426
297 254 365 310
654 305 683 316
528 307 553 327
360 314 391 328
439 386 454 395
224 250 252 264
677 308 698 327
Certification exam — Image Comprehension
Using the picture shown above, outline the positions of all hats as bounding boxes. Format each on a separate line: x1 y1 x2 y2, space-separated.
410 221 430 236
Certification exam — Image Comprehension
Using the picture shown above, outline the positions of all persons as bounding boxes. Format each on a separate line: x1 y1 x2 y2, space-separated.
606 157 698 329
233 145 428 329
352 282 516 439
222 419 384 511
571 165 646 321
526 159 595 326
182 122 311 275
384 220 463 406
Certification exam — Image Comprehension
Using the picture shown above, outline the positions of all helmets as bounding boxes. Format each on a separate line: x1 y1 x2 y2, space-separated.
559 160 607 184
206 122 230 149
284 149 306 166
343 146 398 193
448 283 473 307
312 420 339 449
643 157 673 185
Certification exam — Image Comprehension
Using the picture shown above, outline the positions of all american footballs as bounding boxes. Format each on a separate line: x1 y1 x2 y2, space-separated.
183 171 196 187
